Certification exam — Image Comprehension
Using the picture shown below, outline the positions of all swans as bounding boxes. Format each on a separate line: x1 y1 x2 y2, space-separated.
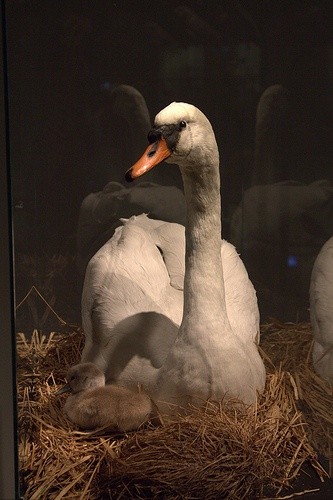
83 101 267 412
56 365 153 431
311 238 333 368
